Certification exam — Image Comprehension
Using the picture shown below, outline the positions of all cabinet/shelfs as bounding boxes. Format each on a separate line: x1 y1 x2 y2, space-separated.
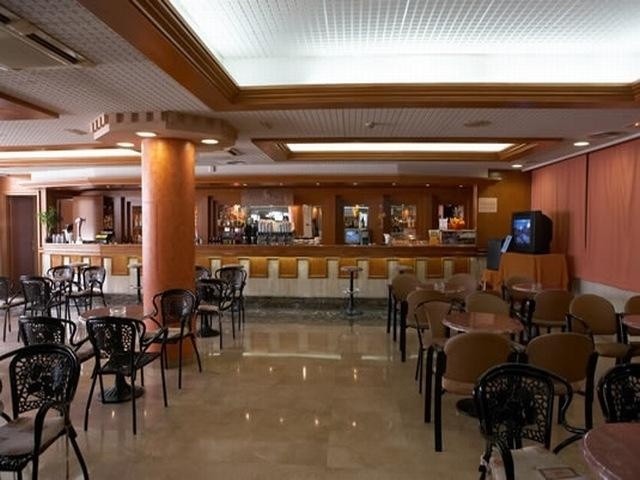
496 252 569 294
42 243 477 297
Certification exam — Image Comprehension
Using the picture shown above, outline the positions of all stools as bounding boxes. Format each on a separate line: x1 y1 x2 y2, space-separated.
392 265 413 274
70 262 90 307
480 269 497 291
341 266 365 316
127 263 143 305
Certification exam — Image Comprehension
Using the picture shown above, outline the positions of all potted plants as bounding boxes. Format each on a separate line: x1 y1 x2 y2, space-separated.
38 206 64 243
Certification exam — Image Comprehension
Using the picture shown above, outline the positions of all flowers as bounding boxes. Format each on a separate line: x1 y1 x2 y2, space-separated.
217 203 248 228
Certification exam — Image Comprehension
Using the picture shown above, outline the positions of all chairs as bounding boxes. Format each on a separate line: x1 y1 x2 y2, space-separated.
399 289 448 362
137 288 202 390
502 275 532 301
509 290 573 342
624 295 640 314
64 266 106 326
0 343 90 480
46 265 80 317
475 362 588 480
597 362 640 423
0 276 28 342
215 267 247 331
525 332 600 430
566 293 631 370
424 330 524 452
386 273 423 342
83 316 168 435
18 315 107 404
465 292 509 315
413 298 465 394
444 273 480 292
17 275 72 342
197 278 237 349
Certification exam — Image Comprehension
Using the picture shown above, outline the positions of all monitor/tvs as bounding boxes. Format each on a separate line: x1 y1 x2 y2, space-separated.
509 210 553 254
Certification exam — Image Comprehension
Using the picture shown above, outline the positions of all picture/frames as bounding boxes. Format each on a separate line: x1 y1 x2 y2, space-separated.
501 235 512 252
478 196 498 213
